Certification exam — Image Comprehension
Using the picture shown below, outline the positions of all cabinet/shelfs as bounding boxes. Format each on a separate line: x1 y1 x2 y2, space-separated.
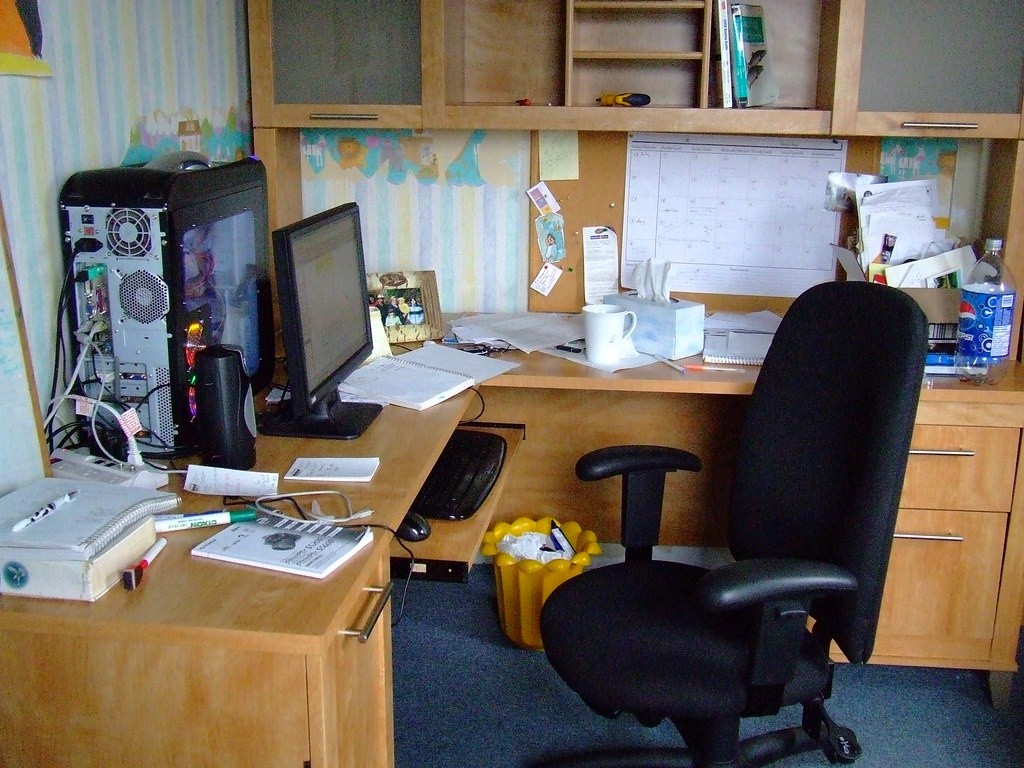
246 0 1024 710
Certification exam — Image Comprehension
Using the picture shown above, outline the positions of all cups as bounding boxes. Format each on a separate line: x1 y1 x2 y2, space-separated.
582 304 637 365
194 343 257 472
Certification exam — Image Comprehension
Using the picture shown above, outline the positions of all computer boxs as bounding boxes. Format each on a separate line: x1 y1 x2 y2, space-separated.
55 149 276 461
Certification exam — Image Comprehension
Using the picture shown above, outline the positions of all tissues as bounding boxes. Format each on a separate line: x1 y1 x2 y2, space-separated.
603 258 705 360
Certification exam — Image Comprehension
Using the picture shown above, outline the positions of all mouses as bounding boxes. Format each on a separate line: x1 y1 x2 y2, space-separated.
393 513 431 541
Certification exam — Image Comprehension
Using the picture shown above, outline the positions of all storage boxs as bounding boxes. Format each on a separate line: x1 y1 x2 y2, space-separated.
834 246 963 343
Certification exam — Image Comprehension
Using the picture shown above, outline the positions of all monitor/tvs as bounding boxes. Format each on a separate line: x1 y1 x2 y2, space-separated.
259 201 382 440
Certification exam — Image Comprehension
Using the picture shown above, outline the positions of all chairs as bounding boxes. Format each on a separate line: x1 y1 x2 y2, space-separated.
518 280 929 768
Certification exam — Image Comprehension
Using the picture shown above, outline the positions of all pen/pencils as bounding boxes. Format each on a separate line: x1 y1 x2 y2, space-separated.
653 353 684 372
689 365 745 373
11 490 79 532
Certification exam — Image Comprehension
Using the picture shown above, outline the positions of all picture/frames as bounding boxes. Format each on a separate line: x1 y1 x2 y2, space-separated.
364 271 445 344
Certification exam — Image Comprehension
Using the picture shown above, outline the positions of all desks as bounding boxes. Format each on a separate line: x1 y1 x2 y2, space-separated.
0 358 489 768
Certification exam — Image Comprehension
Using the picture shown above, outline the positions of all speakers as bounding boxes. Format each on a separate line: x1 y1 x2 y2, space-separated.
195 343 257 472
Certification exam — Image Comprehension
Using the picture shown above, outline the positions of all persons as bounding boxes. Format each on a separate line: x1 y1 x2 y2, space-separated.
368 294 423 326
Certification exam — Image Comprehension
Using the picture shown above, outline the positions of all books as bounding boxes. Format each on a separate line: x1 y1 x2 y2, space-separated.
717 0 777 109
0 476 183 601
884 245 978 343
337 342 522 412
191 509 374 580
701 329 776 365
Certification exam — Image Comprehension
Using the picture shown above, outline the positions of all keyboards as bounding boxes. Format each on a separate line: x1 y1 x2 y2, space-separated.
410 429 506 521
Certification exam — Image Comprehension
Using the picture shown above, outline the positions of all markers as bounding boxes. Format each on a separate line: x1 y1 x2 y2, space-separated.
153 509 259 533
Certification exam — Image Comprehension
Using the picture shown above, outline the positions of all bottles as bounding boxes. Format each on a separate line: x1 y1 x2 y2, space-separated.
954 237 1017 386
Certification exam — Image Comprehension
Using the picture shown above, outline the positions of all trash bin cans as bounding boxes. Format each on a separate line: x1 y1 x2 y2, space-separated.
481 514 603 652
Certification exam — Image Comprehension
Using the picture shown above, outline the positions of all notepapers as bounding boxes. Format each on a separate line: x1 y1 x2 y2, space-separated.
284 458 380 483
